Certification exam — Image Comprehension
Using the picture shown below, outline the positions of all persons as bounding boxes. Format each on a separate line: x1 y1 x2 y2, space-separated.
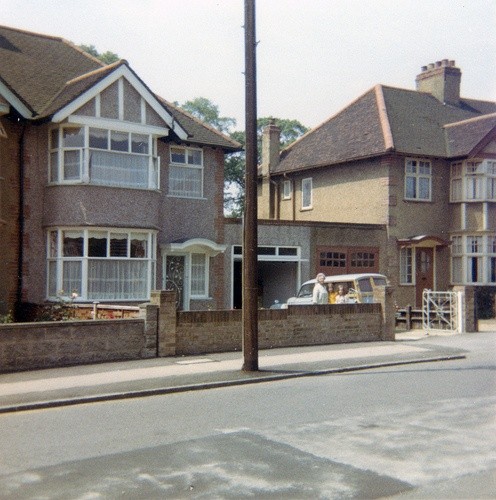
335 284 350 304
312 272 328 304
326 280 338 304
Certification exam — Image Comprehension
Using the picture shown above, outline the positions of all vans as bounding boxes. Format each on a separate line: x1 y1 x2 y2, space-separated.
269 273 400 328
